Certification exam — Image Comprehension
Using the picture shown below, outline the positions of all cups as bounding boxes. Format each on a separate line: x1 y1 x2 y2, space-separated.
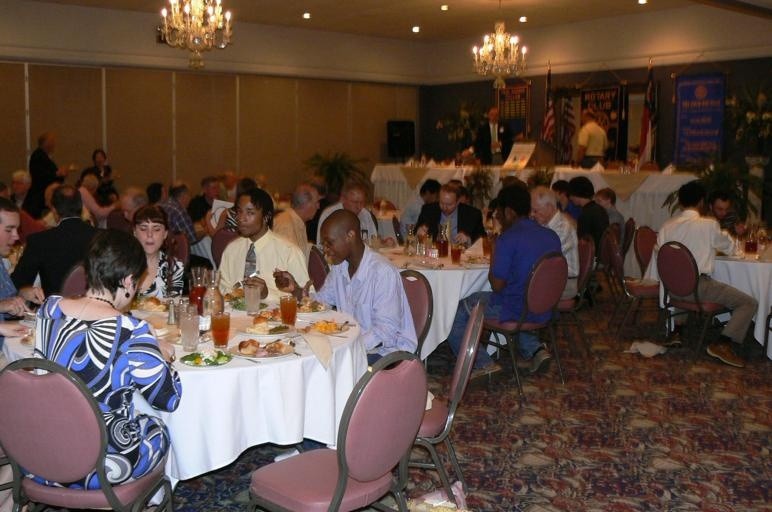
405 223 493 265
176 266 297 352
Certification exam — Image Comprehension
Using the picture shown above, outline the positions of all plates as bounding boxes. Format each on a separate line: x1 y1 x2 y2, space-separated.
132 297 351 368
18 318 36 347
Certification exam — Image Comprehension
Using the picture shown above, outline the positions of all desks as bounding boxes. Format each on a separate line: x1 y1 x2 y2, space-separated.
369 161 701 282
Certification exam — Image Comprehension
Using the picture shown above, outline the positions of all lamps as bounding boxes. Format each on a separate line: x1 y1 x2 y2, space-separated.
159 0 234 69
470 1 529 89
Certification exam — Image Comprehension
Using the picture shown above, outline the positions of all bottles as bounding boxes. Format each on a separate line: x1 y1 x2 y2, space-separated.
746 223 759 254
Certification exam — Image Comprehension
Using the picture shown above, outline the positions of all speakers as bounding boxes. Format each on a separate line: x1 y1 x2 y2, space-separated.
388 121 415 157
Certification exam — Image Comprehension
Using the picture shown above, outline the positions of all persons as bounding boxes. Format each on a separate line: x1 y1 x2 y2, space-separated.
471 103 512 165
23 229 182 512
2 130 625 451
658 182 759 368
573 108 609 168
701 189 747 238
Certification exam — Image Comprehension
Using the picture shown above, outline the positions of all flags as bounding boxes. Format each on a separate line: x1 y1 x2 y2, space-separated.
636 64 658 168
537 68 558 149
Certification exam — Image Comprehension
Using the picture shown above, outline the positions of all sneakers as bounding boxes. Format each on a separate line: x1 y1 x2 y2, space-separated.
529 351 551 375
661 330 685 349
706 342 745 369
470 363 501 382
272 449 300 463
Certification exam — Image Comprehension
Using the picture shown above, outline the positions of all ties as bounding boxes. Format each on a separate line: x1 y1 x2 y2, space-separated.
244 244 257 283
442 216 452 243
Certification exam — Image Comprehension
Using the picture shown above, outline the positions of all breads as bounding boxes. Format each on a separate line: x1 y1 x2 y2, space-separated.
231 288 243 297
238 339 260 355
143 296 167 312
